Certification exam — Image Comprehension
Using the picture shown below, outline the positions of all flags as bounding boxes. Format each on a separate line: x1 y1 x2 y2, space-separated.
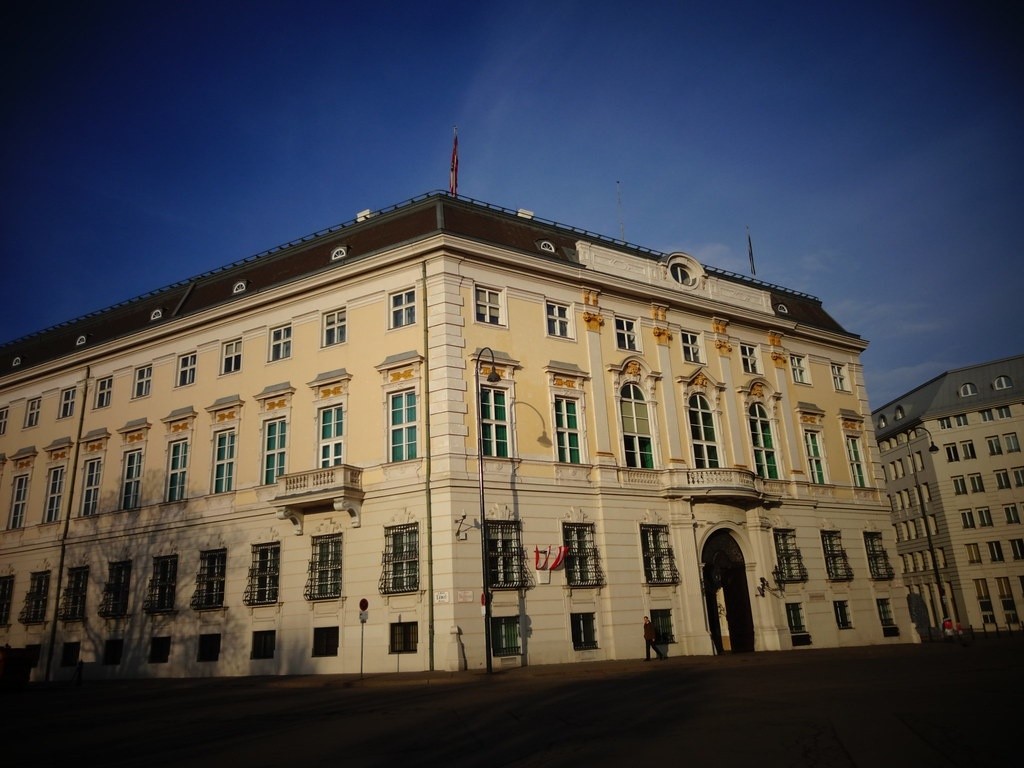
535 546 569 570
449 137 458 197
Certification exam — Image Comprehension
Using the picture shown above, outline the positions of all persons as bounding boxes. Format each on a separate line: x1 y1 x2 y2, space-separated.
643 617 663 662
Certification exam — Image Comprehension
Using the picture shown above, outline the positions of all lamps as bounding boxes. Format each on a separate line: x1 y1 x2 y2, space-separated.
454 509 466 541
759 564 786 598
